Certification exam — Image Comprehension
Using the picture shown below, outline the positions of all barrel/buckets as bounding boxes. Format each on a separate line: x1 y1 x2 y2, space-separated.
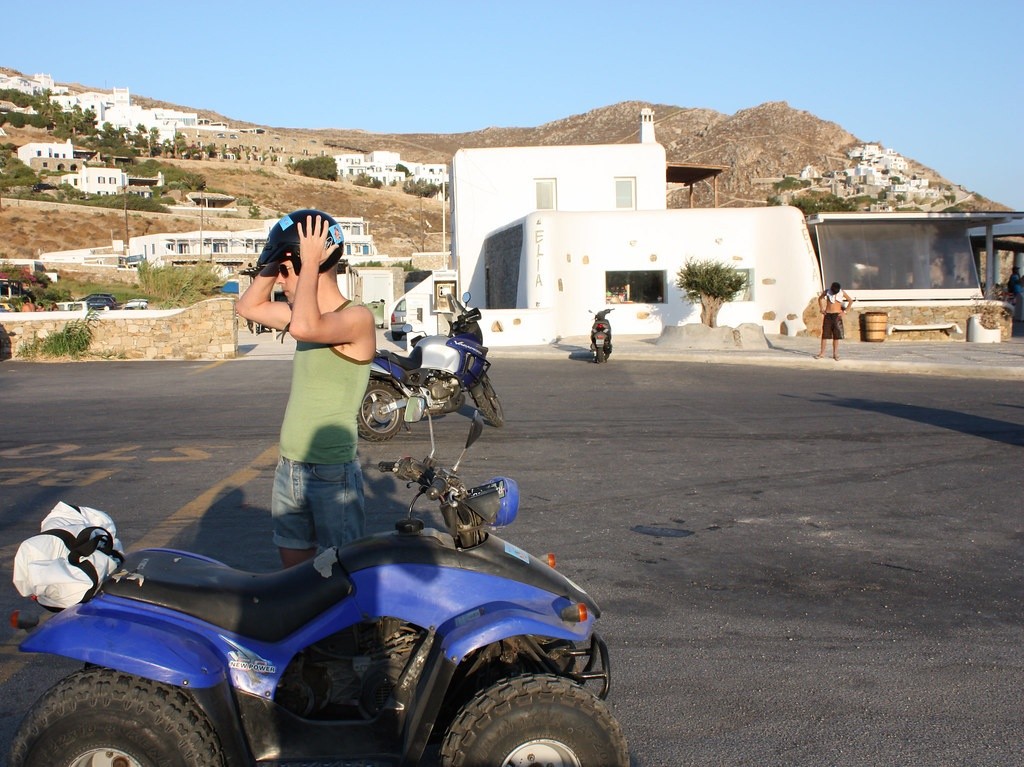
864 312 888 342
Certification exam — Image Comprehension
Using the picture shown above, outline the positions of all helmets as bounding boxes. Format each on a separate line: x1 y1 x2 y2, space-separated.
257 209 344 276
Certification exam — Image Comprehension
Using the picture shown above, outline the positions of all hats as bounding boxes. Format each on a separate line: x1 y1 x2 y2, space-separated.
1013 266 1020 270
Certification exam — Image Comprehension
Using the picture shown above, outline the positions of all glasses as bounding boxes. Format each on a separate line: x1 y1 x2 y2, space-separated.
280 261 294 277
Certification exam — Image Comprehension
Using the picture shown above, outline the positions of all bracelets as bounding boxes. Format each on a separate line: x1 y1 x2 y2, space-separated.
843 310 847 314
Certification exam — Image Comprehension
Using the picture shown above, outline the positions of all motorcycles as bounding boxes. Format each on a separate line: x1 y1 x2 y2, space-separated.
1 391 631 767
356 291 509 443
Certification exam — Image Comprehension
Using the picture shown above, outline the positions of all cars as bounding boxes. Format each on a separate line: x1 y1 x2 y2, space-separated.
125 254 147 268
121 298 149 310
391 297 408 342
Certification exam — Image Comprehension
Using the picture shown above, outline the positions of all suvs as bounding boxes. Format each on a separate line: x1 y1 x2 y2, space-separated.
82 295 116 310
76 293 117 305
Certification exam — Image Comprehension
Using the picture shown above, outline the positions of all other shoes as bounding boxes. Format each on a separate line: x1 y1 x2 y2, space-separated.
815 354 824 359
833 356 841 361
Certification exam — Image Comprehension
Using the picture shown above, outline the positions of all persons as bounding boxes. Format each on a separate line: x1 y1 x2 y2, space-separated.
815 282 853 361
1008 266 1024 298
21 297 45 311
51 302 58 311
236 210 376 567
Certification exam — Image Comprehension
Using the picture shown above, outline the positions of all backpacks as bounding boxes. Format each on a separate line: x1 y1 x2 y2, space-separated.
1008 275 1016 293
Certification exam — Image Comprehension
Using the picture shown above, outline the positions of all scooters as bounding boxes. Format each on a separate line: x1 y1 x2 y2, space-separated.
588 306 616 364
